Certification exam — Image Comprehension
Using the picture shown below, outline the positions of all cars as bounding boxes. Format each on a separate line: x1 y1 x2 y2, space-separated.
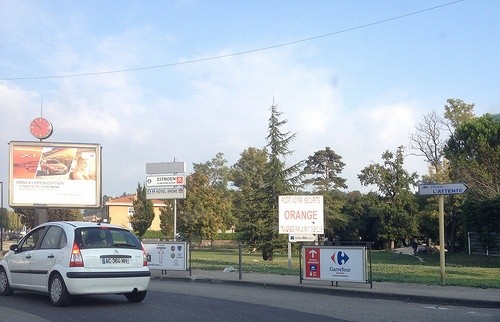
0 221 153 307
